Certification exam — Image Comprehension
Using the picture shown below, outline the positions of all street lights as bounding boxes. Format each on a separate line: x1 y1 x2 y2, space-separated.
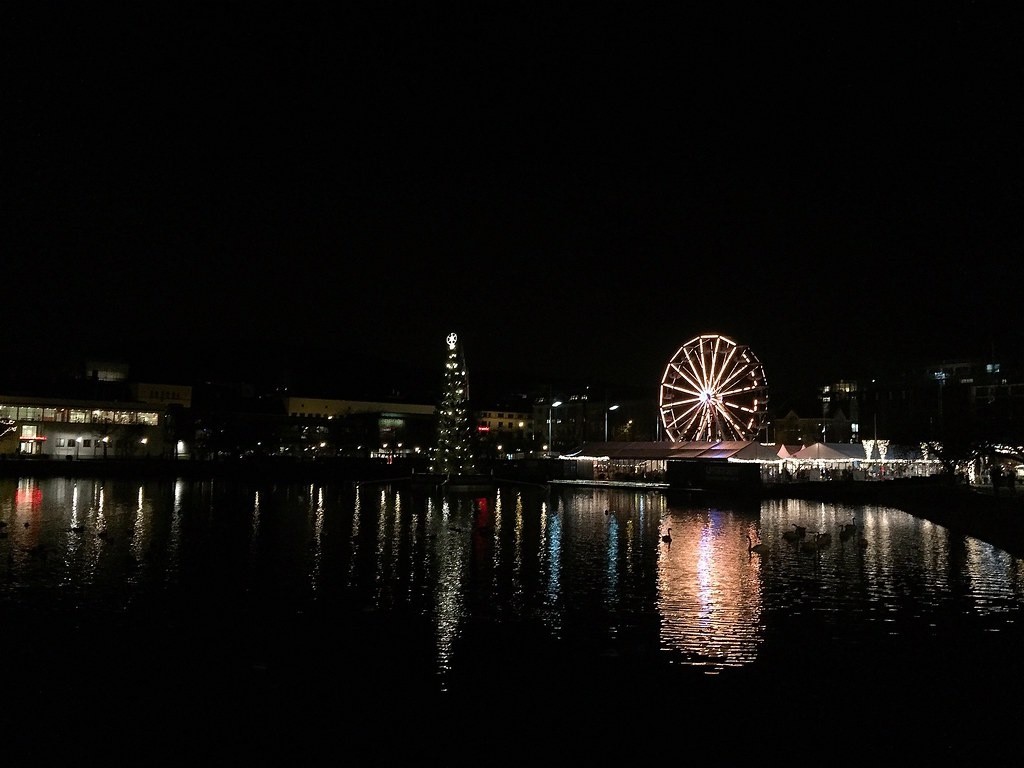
549 401 563 451
604 404 619 443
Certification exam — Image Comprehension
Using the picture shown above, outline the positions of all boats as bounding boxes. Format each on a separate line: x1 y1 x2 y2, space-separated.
78 442 168 475
